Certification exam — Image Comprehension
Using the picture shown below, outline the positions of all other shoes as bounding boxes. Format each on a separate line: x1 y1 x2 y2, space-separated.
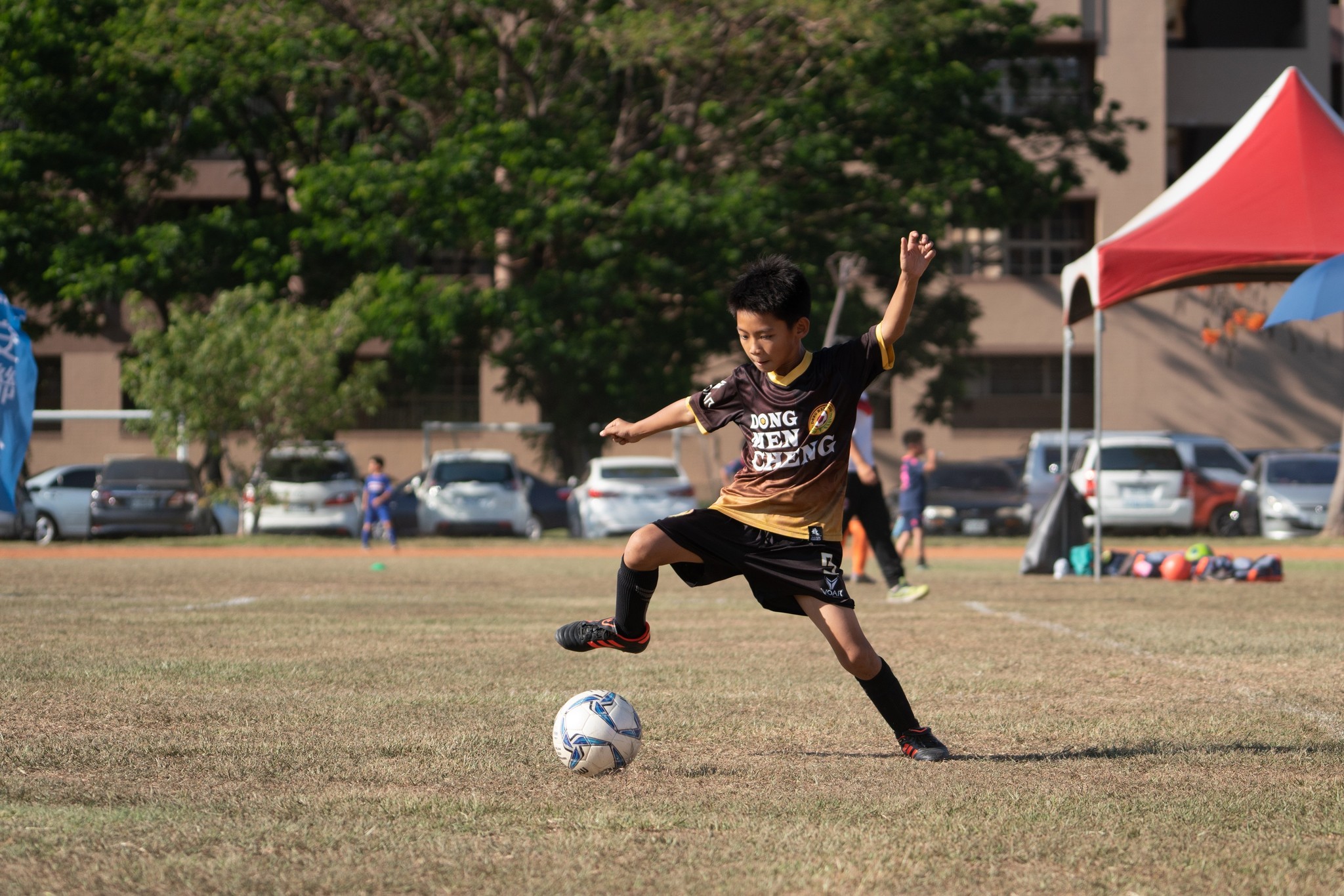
851 574 873 584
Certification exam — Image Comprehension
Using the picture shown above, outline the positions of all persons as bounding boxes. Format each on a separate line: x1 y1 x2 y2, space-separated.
839 372 938 603
553 230 948 762
361 456 397 552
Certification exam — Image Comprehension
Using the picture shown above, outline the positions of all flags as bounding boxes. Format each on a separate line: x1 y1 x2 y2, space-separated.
0 290 38 515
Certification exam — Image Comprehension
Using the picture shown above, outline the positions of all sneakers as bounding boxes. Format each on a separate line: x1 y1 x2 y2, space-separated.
892 724 949 763
555 616 651 653
887 580 930 603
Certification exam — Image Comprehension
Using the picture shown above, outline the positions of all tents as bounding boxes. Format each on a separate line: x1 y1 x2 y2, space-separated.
1060 65 1344 580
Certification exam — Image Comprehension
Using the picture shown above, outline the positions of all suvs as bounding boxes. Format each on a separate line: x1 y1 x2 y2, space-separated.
1070 435 1198 534
411 443 535 538
237 439 366 538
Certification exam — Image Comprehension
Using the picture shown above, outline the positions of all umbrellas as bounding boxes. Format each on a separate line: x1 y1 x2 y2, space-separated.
1261 254 1344 328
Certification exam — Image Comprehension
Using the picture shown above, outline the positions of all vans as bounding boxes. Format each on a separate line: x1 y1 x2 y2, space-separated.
1019 428 1255 530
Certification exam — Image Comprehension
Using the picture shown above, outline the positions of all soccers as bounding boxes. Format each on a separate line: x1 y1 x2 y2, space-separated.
553 691 641 778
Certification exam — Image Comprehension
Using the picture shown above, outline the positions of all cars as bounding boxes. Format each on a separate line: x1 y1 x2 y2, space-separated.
915 455 1029 537
0 463 102 542
86 454 215 534
563 454 699 538
1253 448 1339 539
1181 466 1249 535
